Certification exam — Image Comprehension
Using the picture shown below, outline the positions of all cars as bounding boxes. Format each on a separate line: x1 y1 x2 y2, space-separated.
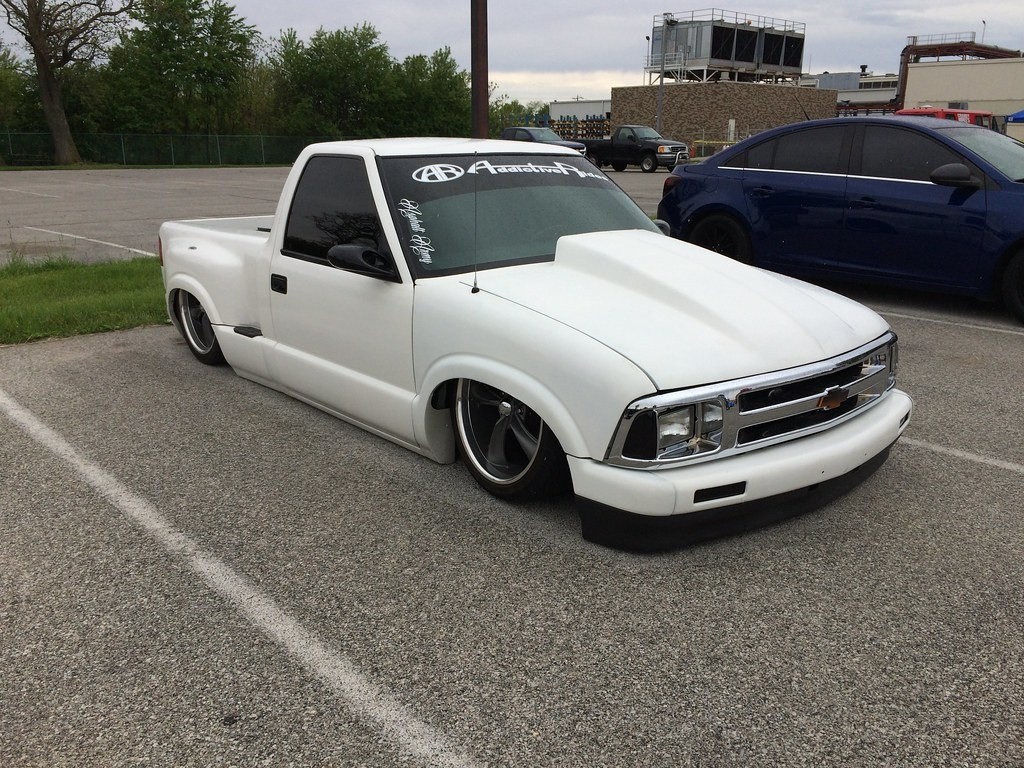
655 114 1024 323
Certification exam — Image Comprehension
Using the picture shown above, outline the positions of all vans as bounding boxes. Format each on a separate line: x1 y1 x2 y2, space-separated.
895 106 1007 136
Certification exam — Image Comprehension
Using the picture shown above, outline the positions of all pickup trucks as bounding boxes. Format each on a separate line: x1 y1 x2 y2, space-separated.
500 126 587 157
157 135 916 553
563 125 691 173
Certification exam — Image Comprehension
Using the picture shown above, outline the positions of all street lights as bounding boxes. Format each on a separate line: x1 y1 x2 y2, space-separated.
977 20 986 59
645 35 650 66
